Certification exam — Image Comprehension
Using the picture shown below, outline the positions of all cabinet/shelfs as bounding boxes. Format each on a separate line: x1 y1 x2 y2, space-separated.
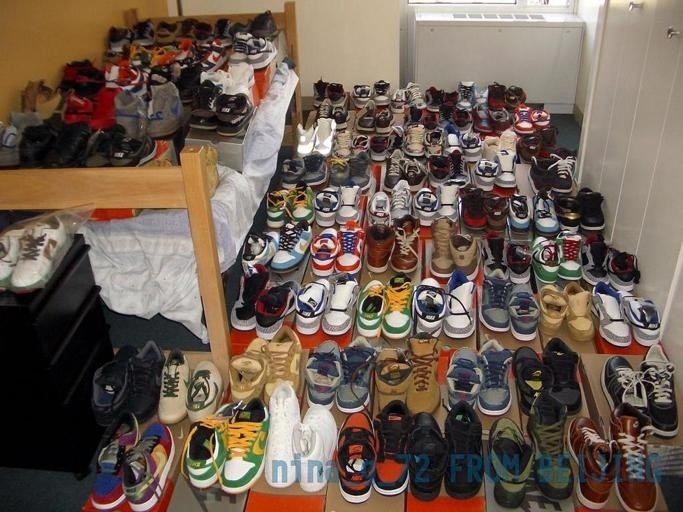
0 234 116 480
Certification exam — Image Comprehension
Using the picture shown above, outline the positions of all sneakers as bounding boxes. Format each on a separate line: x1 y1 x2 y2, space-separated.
0 214 74 294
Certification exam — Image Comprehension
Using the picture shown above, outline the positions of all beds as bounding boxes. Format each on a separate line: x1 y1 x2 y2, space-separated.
0 2 300 374
